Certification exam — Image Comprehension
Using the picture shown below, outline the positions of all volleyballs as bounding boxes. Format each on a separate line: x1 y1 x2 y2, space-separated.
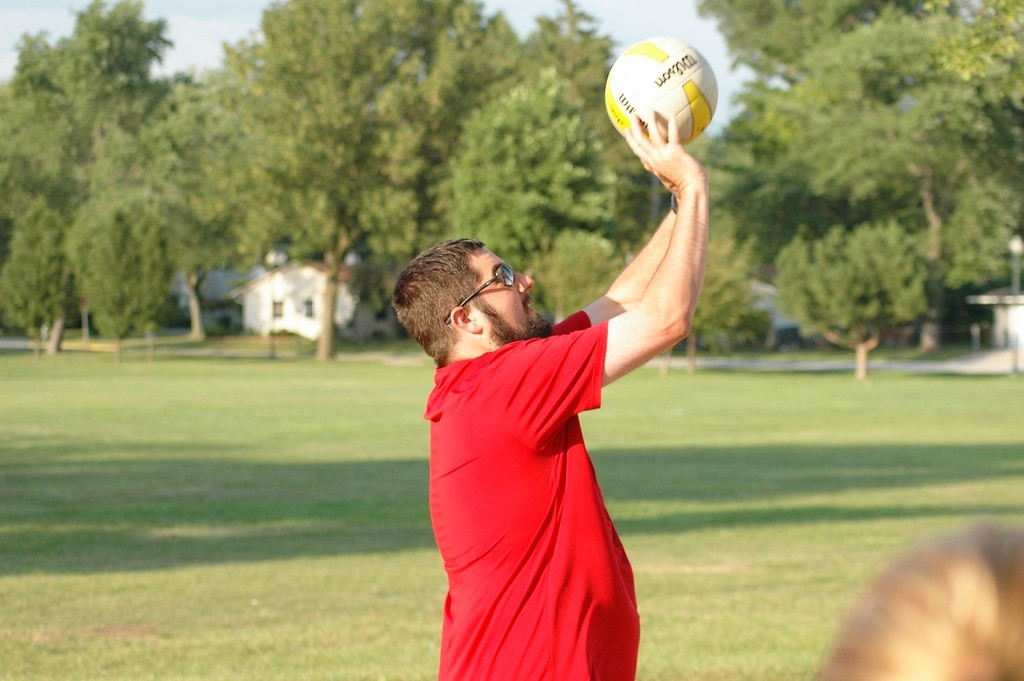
606 39 718 146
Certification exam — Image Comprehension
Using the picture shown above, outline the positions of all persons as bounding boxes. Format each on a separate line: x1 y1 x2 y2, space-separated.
392 110 710 681
817 518 1024 681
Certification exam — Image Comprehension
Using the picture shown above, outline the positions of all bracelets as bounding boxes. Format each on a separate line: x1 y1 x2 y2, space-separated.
670 194 678 216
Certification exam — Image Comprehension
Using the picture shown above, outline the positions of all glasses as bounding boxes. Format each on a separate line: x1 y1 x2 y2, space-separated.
445 263 514 324
